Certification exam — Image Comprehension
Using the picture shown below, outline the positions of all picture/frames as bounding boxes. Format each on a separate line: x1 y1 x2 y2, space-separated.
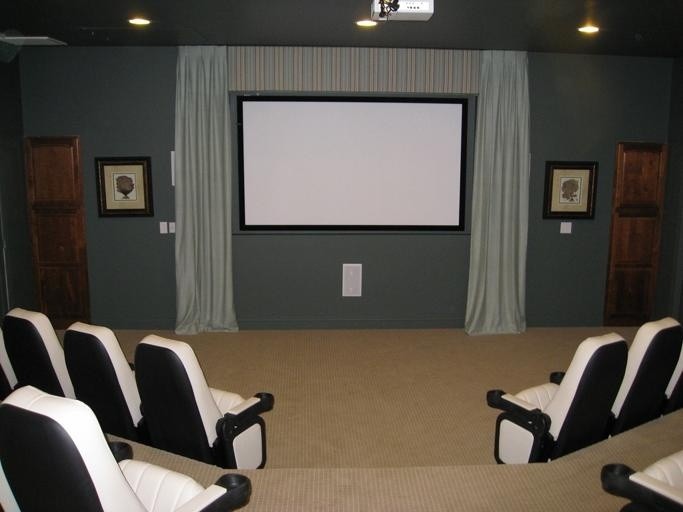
543 161 598 219
93 156 154 219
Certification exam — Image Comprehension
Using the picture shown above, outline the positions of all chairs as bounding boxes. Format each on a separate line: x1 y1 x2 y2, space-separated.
63 321 142 441
1 308 76 401
0 384 254 512
486 316 683 512
134 334 272 470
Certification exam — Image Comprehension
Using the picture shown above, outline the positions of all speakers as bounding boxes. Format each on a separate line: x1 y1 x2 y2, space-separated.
342 263 362 297
171 151 174 185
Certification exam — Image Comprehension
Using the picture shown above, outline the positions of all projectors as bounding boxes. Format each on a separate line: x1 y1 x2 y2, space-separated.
370 0 435 23
